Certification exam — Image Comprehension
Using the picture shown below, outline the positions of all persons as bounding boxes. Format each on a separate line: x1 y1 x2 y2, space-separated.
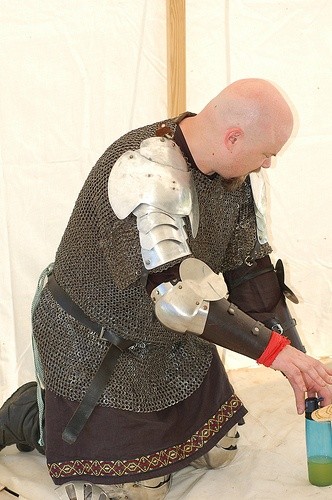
0 78 332 500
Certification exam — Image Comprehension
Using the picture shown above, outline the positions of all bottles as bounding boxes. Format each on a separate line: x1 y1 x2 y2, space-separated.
305 396 332 486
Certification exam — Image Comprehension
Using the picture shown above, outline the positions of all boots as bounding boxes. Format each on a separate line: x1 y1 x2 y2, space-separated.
0 381 42 454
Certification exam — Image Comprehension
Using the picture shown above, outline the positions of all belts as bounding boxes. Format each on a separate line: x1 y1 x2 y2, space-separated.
47 272 136 446
